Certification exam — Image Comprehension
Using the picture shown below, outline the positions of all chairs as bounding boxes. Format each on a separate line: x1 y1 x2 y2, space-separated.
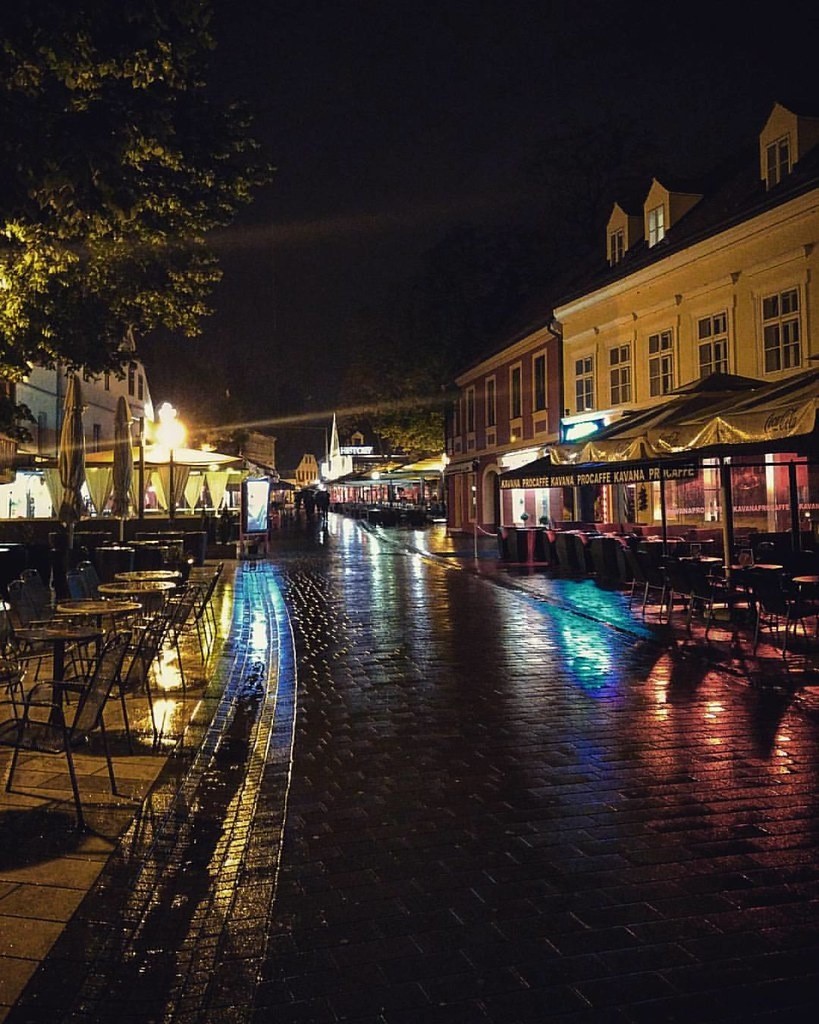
0 508 243 830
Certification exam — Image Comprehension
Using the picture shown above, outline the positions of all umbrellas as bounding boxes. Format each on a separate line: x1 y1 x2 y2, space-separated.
323 453 452 509
30 443 295 516
59 371 90 548
110 396 136 542
499 368 819 643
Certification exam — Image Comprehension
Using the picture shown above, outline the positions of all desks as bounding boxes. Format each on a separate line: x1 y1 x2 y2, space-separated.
496 524 819 661
330 501 445 528
